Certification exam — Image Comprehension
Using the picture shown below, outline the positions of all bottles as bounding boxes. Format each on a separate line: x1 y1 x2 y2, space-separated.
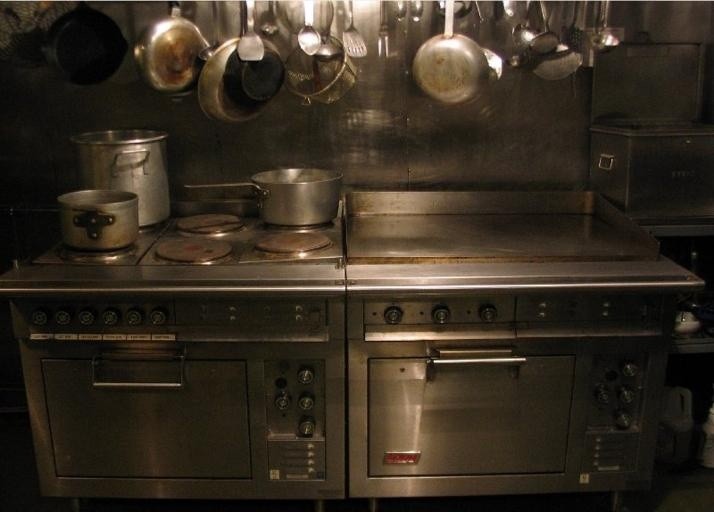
700 407 714 468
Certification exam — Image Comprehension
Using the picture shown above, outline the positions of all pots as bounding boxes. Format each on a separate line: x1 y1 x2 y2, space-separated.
411 2 491 111
43 2 129 87
68 128 173 229
132 2 287 127
183 166 344 227
8 187 140 251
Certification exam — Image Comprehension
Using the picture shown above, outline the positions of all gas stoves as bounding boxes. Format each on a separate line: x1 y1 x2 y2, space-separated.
30 202 341 266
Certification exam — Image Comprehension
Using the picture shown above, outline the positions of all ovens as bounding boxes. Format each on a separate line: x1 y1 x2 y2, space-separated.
344 287 684 500
1 277 348 501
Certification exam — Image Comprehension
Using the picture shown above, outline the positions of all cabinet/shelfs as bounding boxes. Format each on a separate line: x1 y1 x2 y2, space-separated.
599 196 714 497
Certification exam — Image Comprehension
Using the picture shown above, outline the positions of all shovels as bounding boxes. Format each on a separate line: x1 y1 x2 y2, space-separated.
236 0 264 61
342 0 368 58
